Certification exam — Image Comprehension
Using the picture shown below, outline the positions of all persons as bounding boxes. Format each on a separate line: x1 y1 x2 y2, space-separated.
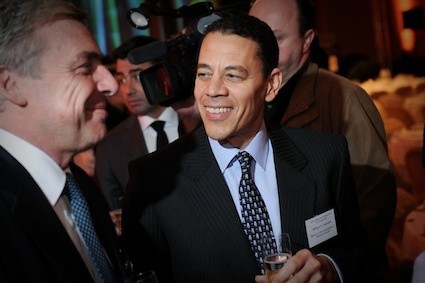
69 32 204 243
249 0 395 281
121 14 360 282
0 1 122 283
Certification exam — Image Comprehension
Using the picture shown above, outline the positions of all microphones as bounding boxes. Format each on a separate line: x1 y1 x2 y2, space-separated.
126 39 176 65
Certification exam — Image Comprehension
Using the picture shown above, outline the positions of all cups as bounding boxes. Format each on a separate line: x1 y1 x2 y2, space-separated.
264 233 292 283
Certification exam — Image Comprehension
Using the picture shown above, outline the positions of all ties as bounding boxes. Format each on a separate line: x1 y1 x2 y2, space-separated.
62 172 118 283
235 151 280 275
150 120 171 151
